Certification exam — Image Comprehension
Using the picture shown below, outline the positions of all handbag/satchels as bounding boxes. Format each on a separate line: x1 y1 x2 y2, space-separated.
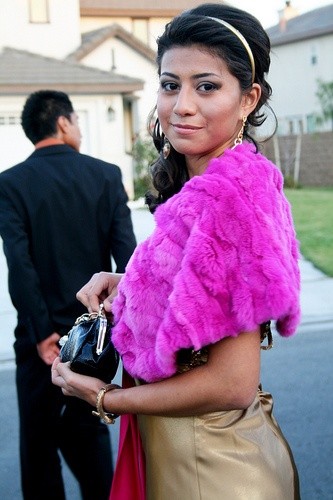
58 303 120 384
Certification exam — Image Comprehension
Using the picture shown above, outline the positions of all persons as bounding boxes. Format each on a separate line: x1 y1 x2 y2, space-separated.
50 2 305 499
0 89 139 499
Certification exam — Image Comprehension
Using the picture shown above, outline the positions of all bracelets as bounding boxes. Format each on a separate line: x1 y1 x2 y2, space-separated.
90 383 126 425
